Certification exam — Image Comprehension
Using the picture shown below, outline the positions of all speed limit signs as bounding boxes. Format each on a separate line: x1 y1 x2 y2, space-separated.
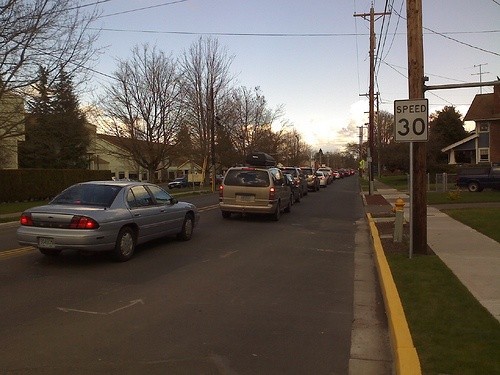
394 98 429 143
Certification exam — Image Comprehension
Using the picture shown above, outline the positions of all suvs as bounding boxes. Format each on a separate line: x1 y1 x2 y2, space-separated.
457 161 500 191
219 167 295 218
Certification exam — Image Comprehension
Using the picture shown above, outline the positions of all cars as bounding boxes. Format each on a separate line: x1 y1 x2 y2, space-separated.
15 181 200 261
283 166 355 202
169 177 186 189
